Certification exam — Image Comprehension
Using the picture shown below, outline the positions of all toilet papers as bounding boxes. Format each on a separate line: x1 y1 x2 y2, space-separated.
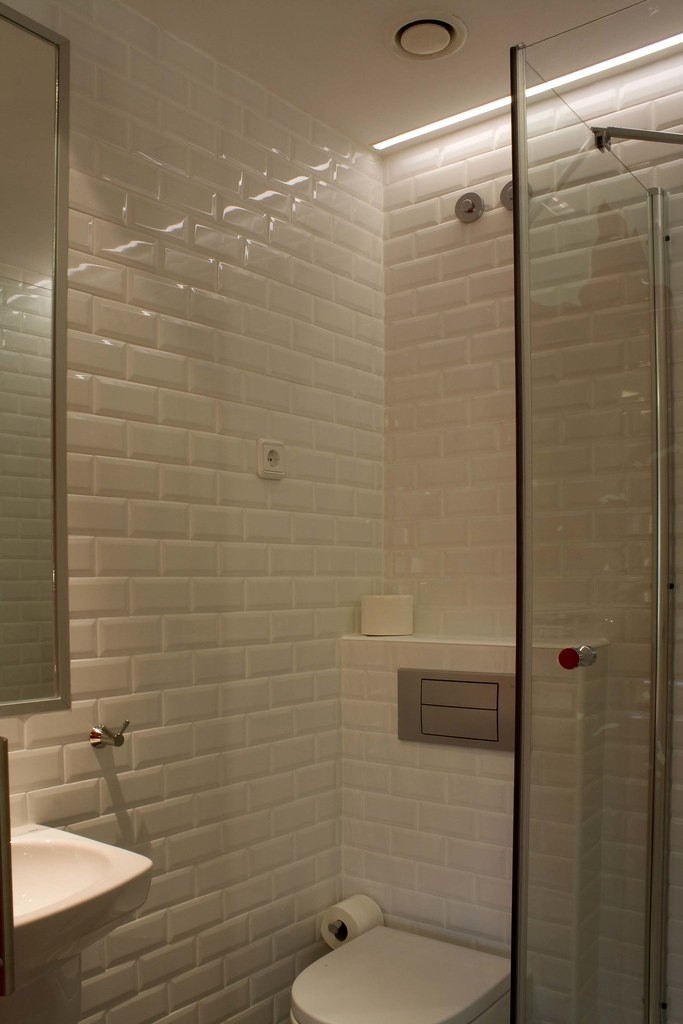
320 893 384 950
359 594 414 637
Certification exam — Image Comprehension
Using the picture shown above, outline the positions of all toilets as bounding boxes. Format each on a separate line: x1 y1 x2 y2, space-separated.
287 925 509 1024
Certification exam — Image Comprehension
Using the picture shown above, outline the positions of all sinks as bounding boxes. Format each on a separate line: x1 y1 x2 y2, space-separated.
0 821 154 982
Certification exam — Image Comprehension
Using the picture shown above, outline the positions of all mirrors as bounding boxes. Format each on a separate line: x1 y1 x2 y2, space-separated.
0 3 71 716
